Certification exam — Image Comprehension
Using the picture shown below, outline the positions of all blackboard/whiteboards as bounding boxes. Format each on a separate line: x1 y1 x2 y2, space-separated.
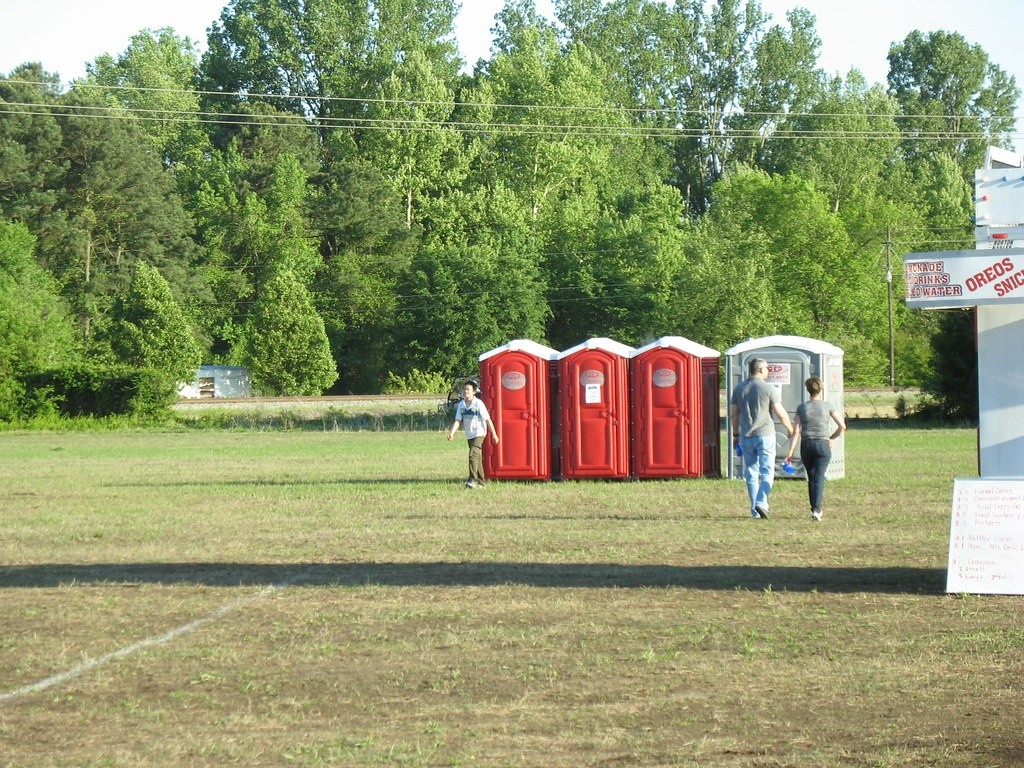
944 477 1024 595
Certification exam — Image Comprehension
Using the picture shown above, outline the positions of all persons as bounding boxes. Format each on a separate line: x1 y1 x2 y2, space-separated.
446 381 499 490
784 378 847 522
730 358 794 520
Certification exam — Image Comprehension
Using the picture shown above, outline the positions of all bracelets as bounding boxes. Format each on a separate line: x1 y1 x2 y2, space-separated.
788 449 794 454
733 432 739 437
787 429 794 433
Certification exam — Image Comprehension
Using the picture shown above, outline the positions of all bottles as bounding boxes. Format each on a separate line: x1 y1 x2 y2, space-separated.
735 444 741 456
781 461 796 475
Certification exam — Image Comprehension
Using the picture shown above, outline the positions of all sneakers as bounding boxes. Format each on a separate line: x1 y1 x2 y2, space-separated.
750 507 759 518
467 479 475 488
477 483 484 489
755 504 768 518
811 508 822 521
